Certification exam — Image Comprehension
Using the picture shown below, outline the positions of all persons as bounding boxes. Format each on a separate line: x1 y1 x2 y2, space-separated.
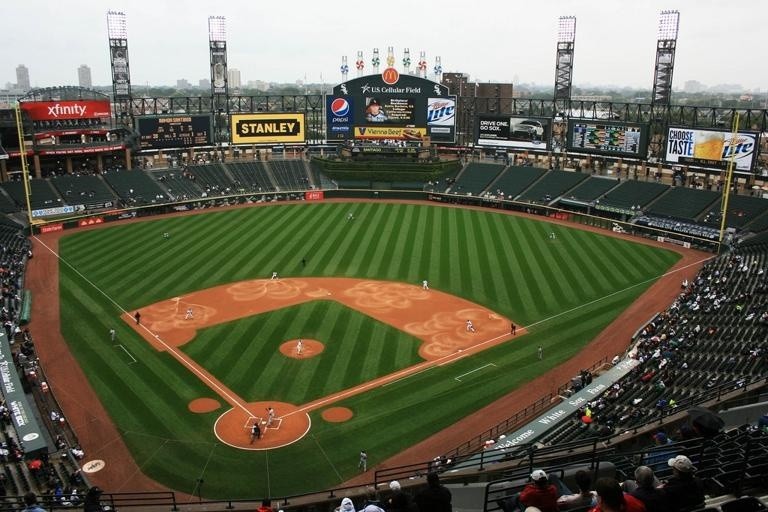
367 99 389 121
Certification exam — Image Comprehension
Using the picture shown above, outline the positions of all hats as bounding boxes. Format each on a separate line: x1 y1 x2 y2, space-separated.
530 469 549 483
653 433 668 445
668 455 694 474
657 400 667 407
389 480 402 493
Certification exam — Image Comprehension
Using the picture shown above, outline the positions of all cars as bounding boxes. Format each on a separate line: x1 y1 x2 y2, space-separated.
513 119 545 142
556 108 620 120
